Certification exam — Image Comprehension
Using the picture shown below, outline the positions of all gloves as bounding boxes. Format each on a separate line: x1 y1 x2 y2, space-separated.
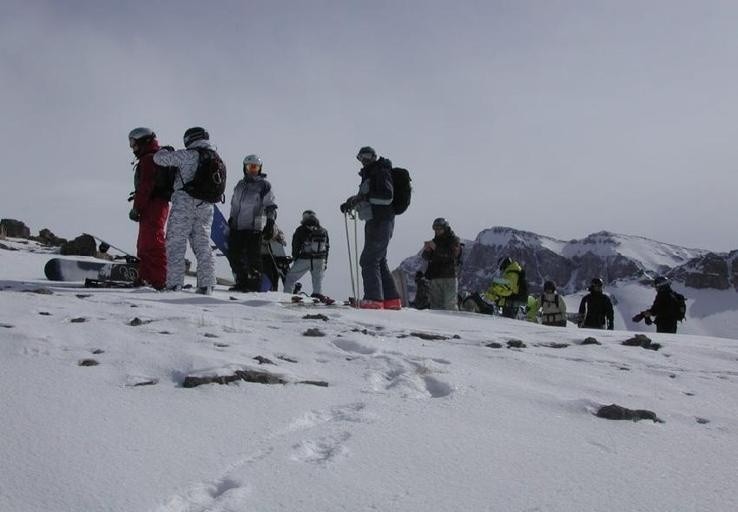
263 219 274 240
129 209 140 222
340 196 360 213
632 312 653 325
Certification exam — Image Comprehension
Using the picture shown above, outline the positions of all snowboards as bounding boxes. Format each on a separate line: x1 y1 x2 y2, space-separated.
210 204 272 293
45 257 140 282
82 232 139 260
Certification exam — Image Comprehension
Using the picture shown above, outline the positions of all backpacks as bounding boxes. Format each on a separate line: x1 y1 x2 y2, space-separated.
160 145 179 202
472 292 494 314
184 147 227 204
391 166 412 215
305 225 328 256
519 269 528 306
673 290 687 321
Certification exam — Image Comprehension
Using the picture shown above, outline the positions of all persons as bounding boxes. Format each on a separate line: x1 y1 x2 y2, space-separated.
578 278 614 330
261 230 302 294
340 146 402 309
153 126 216 294
491 255 528 319
536 280 567 326
422 217 460 311
642 276 677 333
228 155 277 294
129 128 175 290
408 271 430 310
283 210 330 295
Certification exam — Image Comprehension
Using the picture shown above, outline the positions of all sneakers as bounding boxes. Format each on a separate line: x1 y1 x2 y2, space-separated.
360 297 383 308
196 285 213 296
130 278 182 292
228 278 261 291
383 294 402 309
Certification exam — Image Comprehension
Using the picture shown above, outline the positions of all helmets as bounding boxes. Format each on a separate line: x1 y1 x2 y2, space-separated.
243 155 263 176
651 276 671 292
544 280 556 293
129 128 156 152
432 217 449 232
458 287 470 300
588 278 603 293
184 126 209 147
497 256 512 271
357 146 377 165
